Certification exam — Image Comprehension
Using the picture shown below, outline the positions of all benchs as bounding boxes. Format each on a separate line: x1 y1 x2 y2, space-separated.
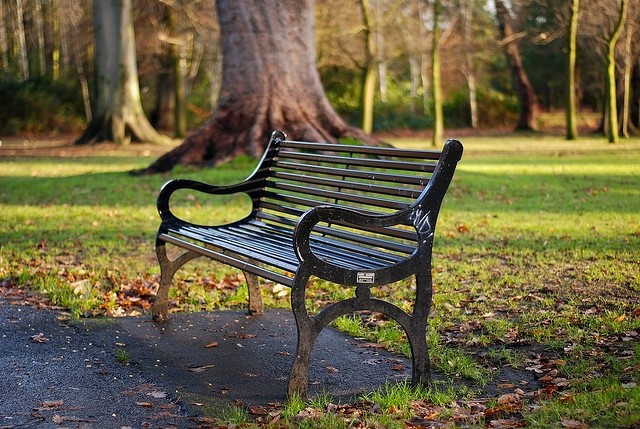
152 131 463 401
